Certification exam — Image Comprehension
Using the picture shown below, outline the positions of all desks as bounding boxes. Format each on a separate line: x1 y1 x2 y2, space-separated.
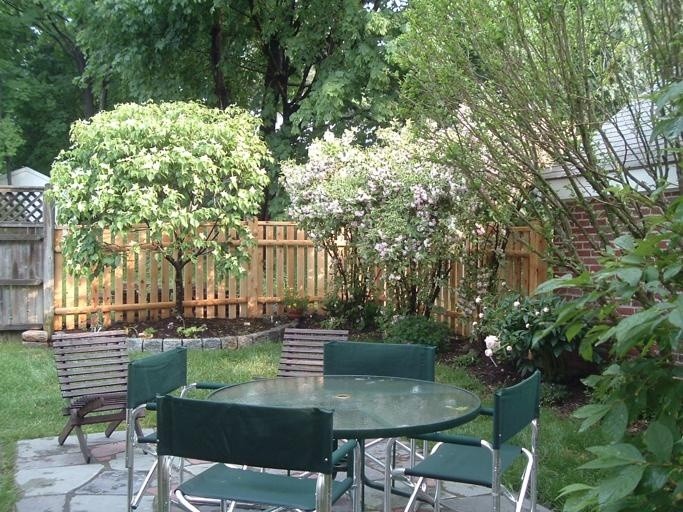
202 374 482 512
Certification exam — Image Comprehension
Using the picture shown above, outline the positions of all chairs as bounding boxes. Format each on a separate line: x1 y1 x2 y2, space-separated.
251 327 348 380
156 393 363 512
318 339 437 509
50 330 148 463
128 342 249 510
384 370 542 512
